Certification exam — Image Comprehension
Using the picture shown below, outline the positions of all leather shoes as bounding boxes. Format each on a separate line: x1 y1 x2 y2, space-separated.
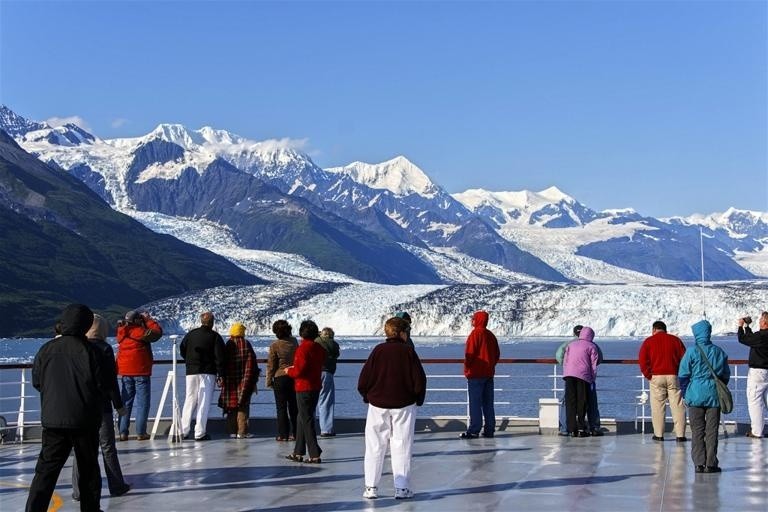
138 433 149 440
578 432 589 437
592 431 604 436
677 437 686 442
704 467 721 473
197 433 210 440
652 436 664 441
746 432 762 437
120 434 128 441
695 465 705 473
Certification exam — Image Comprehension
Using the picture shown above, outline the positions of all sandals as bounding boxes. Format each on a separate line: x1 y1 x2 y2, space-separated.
285 454 303 462
304 456 321 463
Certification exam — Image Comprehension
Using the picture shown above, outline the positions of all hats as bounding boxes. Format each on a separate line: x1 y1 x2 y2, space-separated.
396 312 411 323
230 323 245 337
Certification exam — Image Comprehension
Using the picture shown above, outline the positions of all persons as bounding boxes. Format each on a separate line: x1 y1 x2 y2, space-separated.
265 319 341 441
676 319 732 474
24 303 107 512
543 301 768 457
355 316 427 499
283 317 328 464
396 311 415 349
180 313 258 440
71 313 162 501
458 310 503 441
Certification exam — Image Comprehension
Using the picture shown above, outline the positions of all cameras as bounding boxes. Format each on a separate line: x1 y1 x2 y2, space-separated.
116 315 148 328
743 315 753 325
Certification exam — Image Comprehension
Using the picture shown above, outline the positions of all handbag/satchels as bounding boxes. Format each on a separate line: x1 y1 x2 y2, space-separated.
716 379 733 413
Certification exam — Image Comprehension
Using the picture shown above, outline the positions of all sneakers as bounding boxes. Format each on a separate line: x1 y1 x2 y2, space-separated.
363 486 378 498
460 432 476 438
395 488 415 499
